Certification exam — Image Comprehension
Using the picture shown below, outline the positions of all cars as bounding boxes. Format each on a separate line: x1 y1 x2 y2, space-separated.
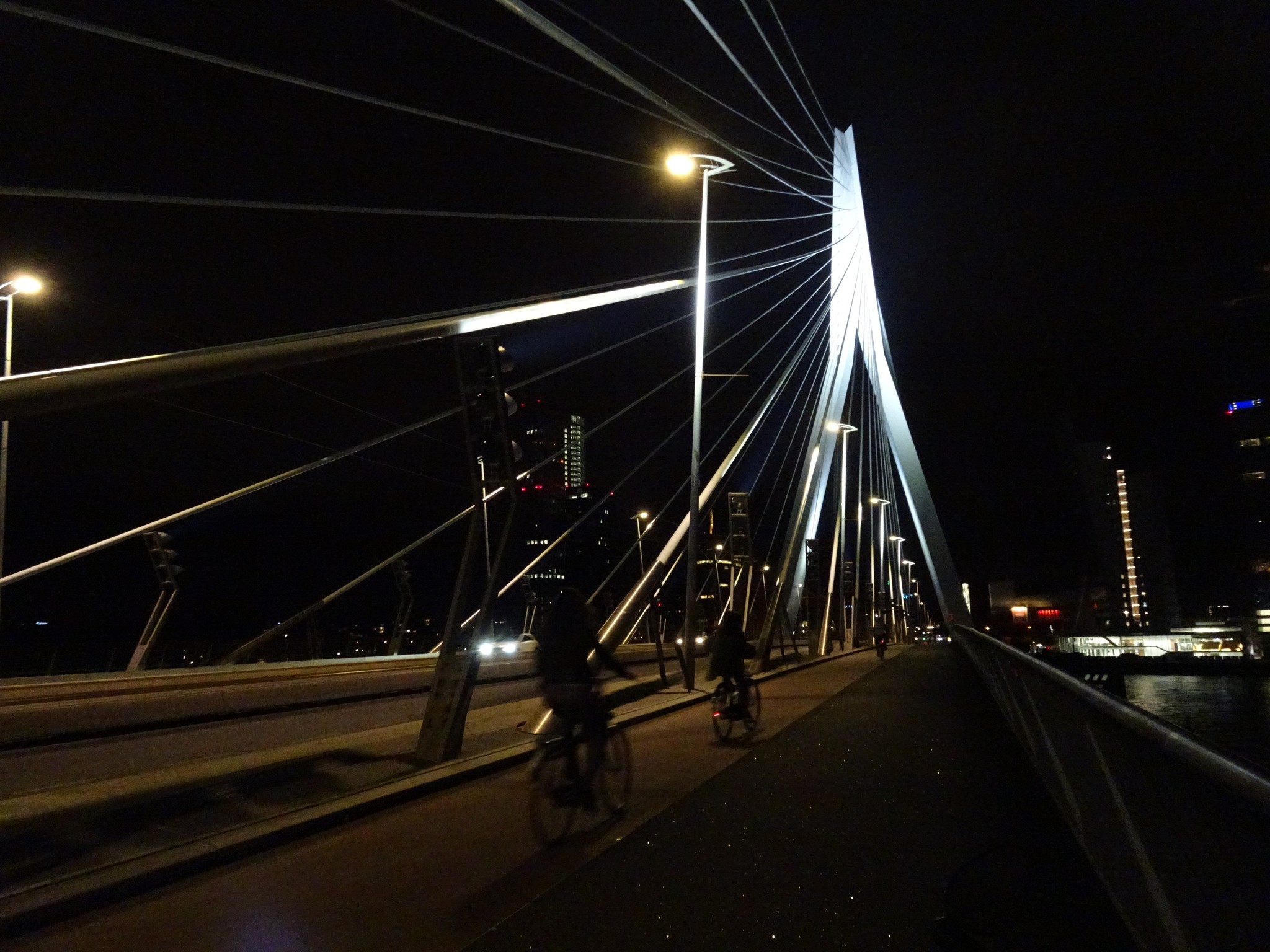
482 633 541 659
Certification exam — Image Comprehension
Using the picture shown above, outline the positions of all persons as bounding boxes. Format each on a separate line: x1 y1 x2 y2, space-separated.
711 612 756 729
873 618 892 656
532 566 637 803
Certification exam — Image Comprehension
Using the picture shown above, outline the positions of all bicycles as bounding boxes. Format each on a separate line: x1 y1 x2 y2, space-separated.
710 674 763 742
877 638 887 661
521 674 636 848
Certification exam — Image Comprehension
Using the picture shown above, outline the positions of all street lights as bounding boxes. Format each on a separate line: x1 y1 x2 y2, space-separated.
889 534 906 643
653 137 740 690
823 420 859 651
902 559 916 643
630 509 651 643
714 543 724 613
920 602 926 620
868 497 891 648
0 262 57 577
914 593 922 625
911 578 919 618
762 564 771 611
